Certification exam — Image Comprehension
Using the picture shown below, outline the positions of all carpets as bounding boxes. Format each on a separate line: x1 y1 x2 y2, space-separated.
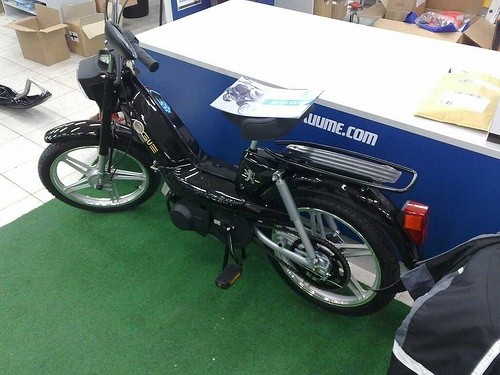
0 145 411 375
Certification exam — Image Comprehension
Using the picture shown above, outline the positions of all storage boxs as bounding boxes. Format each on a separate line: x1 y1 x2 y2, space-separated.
5 3 70 66
63 3 124 57
313 0 348 20
355 0 497 49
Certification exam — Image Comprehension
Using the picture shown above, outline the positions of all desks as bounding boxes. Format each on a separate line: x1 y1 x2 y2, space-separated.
133 0 500 261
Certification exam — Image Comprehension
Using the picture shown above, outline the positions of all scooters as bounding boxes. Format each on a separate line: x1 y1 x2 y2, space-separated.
36 0 432 320
